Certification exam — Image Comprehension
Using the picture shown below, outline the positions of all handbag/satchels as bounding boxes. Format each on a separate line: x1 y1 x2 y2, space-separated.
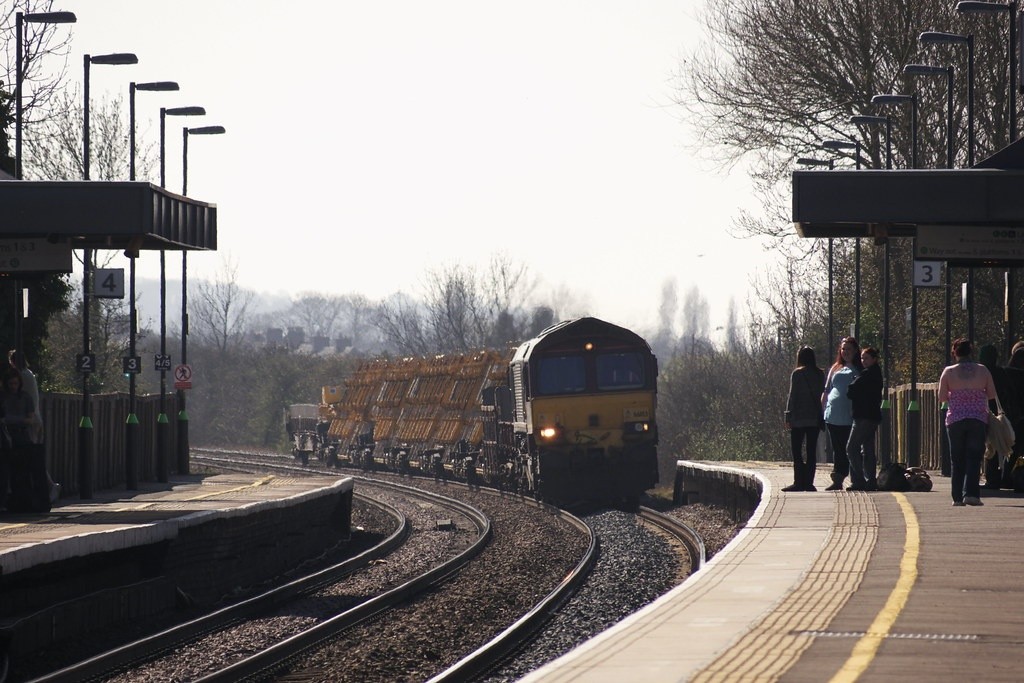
985 393 1015 454
818 410 826 432
875 464 933 492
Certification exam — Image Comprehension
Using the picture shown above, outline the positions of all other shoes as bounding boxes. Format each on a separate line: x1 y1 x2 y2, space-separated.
964 495 983 505
846 480 867 492
979 481 1000 490
951 499 964 505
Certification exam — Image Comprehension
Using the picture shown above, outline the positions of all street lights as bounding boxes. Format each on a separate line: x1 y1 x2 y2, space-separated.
125 81 181 491
902 66 954 477
80 53 138 500
871 95 920 469
954 1 1019 370
796 157 835 462
155 105 207 484
822 142 861 340
916 31 976 340
852 115 892 469
15 10 76 458
176 125 226 476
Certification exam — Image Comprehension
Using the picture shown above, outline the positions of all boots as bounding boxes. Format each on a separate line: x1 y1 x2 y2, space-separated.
805 463 817 492
781 462 806 491
825 473 845 491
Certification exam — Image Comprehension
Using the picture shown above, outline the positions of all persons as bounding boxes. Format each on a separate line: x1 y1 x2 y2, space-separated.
938 338 996 507
979 340 1024 494
820 336 860 489
780 346 826 491
0 350 61 513
846 348 883 490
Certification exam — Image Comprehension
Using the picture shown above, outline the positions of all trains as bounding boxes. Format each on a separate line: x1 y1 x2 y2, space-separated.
284 316 660 513
